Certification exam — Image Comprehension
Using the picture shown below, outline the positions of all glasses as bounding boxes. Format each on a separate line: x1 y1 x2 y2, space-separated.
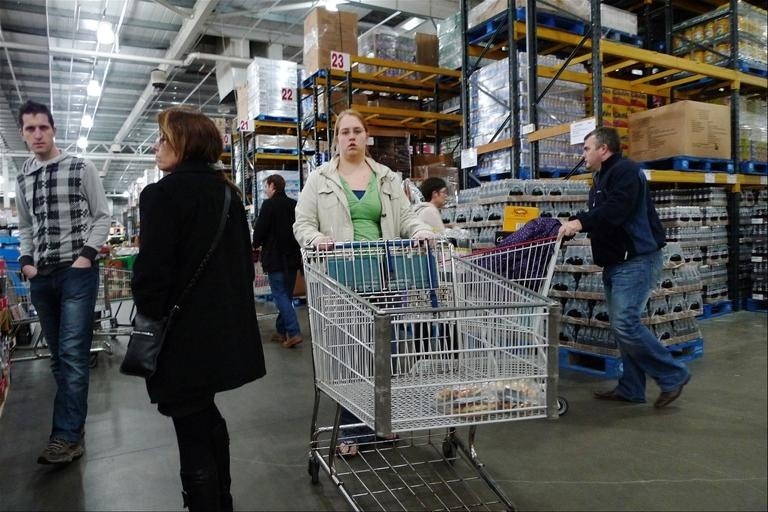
159 137 168 145
436 189 448 195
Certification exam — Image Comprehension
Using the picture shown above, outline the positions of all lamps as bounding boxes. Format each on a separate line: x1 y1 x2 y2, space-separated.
76 132 89 149
79 112 95 131
94 7 118 47
85 74 103 100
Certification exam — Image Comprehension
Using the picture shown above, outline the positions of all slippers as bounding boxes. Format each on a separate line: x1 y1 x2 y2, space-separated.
337 436 360 458
378 431 398 443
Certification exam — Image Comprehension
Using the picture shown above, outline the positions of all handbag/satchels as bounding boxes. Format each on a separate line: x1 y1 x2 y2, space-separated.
118 311 172 380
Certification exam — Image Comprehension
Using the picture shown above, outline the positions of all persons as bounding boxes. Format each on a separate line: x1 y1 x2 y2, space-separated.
291 110 436 457
126 109 267 511
558 127 692 409
15 100 111 464
253 174 303 347
414 178 448 235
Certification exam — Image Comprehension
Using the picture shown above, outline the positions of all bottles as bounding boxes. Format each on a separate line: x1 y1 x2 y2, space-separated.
441 177 768 350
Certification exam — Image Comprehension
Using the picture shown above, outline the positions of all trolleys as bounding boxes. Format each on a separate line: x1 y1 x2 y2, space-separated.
297 234 565 510
246 246 279 322
3 256 113 369
441 228 574 422
88 261 144 342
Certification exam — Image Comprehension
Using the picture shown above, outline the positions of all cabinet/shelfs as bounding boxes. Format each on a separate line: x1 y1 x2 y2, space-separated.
302 58 460 148
460 0 768 192
243 117 310 171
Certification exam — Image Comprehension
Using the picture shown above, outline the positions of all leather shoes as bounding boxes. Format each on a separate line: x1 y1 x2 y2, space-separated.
652 369 693 409
593 383 648 404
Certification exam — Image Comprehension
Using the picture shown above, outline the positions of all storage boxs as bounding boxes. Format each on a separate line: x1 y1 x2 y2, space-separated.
304 6 358 73
413 30 440 80
627 99 732 161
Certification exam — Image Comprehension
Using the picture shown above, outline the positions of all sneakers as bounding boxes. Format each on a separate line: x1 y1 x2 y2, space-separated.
282 333 303 348
36 433 88 466
271 333 286 342
